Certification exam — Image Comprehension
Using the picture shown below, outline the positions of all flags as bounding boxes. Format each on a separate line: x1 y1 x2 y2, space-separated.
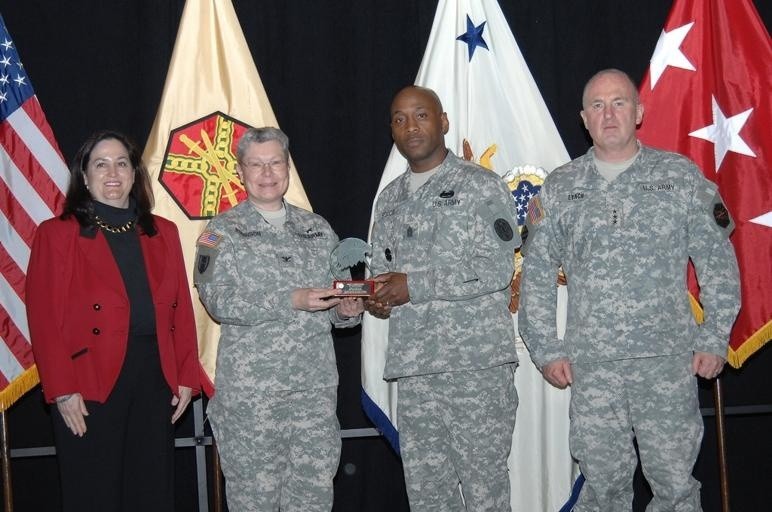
634 0 772 370
0 14 72 413
135 0 315 400
358 0 586 512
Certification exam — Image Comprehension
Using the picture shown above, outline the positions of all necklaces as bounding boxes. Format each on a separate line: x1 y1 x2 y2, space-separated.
94 215 135 234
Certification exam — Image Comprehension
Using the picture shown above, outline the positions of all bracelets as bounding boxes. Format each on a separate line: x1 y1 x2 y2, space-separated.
55 393 73 403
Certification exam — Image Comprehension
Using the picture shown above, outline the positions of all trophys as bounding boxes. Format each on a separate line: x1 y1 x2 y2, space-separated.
329 238 375 297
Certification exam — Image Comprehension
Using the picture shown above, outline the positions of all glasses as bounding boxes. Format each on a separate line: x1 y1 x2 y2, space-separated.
241 161 287 171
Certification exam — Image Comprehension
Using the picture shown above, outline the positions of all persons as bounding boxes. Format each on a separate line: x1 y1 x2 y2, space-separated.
360 84 526 510
520 68 744 512
24 129 203 512
192 127 365 512
